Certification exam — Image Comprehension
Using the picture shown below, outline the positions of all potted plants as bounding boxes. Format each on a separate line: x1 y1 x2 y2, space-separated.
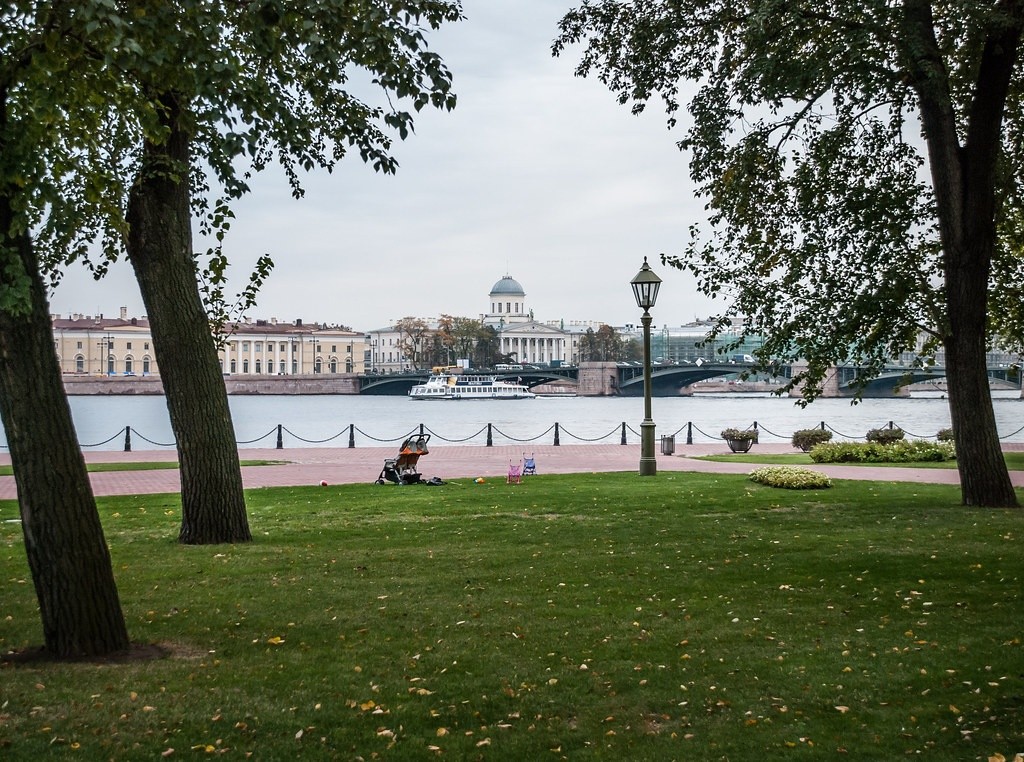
722 428 758 453
865 426 904 445
791 429 833 453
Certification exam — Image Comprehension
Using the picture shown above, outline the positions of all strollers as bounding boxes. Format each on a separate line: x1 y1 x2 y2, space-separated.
374 433 431 488
506 458 524 485
522 451 537 476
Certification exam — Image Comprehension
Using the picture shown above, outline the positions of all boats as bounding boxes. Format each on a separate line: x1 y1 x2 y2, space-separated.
408 373 536 401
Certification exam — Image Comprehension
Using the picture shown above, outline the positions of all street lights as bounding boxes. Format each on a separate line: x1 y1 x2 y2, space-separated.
630 255 664 478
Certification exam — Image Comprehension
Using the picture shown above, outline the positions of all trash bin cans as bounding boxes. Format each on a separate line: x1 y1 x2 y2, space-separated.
661 435 676 456
